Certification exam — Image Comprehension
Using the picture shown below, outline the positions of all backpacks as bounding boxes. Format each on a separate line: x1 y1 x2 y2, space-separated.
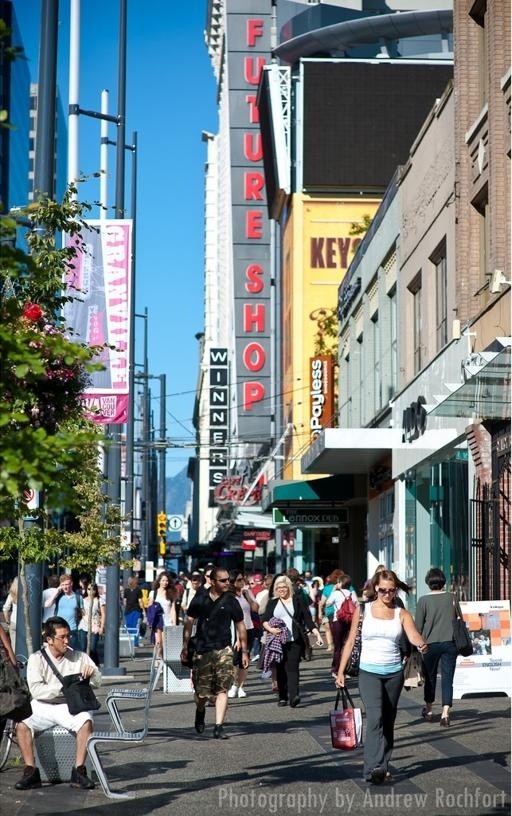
336 589 357 623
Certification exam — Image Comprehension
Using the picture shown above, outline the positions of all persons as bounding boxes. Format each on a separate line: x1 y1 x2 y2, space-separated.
147 571 177 656
329 571 429 784
15 617 102 789
79 584 105 669
74 572 90 596
123 576 144 647
242 573 275 661
415 569 474 727
260 576 324 709
326 576 360 679
45 575 85 651
3 576 19 656
141 583 153 627
288 568 324 632
0 624 34 748
42 573 58 629
227 570 259 698
173 571 211 613
181 567 249 739
319 569 354 651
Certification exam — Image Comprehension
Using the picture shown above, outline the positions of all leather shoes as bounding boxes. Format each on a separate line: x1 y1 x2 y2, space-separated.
277 701 287 707
195 707 206 734
212 724 229 739
290 696 300 708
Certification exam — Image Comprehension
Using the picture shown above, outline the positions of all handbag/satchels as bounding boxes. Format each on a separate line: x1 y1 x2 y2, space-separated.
98 597 105 634
152 590 158 604
341 604 365 677
0 647 33 723
452 595 474 657
40 648 101 716
329 686 363 750
179 592 232 668
278 597 313 662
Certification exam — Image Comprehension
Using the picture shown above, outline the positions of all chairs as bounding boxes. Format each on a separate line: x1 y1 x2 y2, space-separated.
33 728 92 783
105 645 158 742
87 671 154 797
164 626 196 693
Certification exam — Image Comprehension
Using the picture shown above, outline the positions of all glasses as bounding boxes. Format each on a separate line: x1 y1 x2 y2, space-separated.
375 585 398 593
205 574 210 578
217 576 230 582
87 587 96 590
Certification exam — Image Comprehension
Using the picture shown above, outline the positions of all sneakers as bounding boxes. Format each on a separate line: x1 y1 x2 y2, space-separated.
69 765 96 790
238 687 247 698
261 670 272 680
227 684 238 698
370 766 387 786
14 766 42 789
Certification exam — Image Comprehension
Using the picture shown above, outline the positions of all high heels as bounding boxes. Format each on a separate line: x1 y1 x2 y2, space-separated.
440 716 451 727
422 707 433 721
